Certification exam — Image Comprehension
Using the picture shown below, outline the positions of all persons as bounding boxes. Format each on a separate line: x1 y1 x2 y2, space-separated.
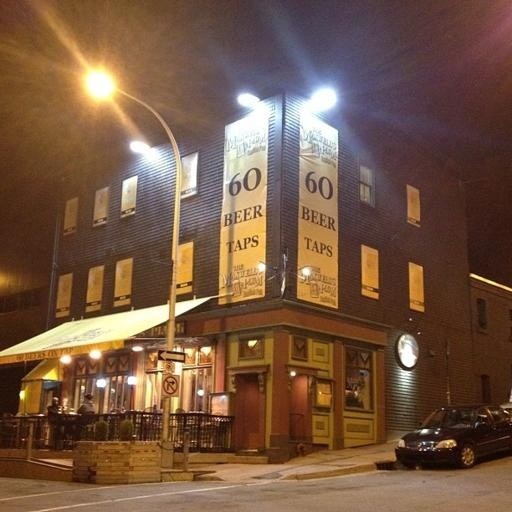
77 393 97 414
47 396 62 414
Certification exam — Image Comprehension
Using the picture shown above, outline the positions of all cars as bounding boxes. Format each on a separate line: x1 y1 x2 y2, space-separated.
395 402 511 470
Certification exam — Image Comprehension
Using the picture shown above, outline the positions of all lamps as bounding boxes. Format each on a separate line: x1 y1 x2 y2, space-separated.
256 246 315 300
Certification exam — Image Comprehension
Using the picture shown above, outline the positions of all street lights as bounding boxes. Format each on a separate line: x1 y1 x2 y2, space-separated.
82 64 182 470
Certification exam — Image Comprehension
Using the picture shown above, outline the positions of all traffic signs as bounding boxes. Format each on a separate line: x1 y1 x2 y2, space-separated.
157 349 185 364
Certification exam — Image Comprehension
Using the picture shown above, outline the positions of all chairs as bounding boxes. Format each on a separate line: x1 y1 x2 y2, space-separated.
1 408 229 452
